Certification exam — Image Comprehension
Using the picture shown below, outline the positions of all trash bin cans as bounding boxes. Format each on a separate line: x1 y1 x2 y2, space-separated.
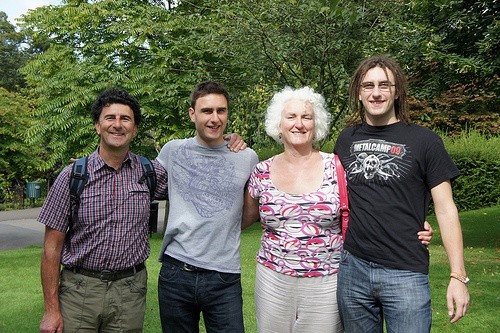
27 182 41 198
150 203 158 234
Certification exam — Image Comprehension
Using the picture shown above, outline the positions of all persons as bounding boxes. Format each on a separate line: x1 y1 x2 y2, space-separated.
36 88 248 333
241 84 434 333
70 80 260 333
333 56 471 333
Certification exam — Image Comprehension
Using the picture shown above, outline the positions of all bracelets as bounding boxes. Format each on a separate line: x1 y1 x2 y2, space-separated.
450 272 469 285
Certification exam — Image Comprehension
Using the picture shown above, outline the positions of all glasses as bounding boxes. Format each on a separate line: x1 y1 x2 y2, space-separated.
360 81 397 92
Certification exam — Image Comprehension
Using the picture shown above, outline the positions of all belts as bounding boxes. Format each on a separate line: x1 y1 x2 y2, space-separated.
66 262 144 282
162 254 203 272
